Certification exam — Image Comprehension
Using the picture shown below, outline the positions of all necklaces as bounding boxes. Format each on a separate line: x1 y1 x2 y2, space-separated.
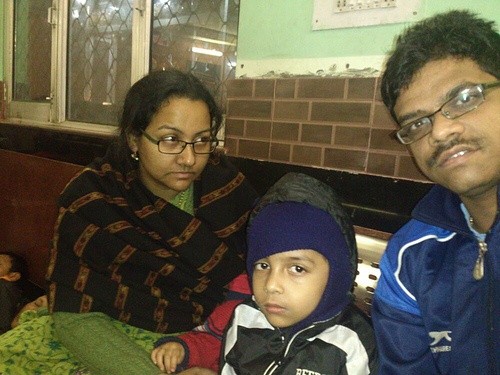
469 216 474 228
179 191 185 208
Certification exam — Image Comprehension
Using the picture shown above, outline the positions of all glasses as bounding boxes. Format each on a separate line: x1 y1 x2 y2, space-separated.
138 125 219 155
387 78 500 146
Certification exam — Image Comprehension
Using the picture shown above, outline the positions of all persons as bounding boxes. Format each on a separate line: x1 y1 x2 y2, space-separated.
149 173 381 375
371 9 500 374
48 68 265 334
0 251 27 336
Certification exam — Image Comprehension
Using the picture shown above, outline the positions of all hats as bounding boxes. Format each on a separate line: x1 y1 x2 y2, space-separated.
245 201 353 333
